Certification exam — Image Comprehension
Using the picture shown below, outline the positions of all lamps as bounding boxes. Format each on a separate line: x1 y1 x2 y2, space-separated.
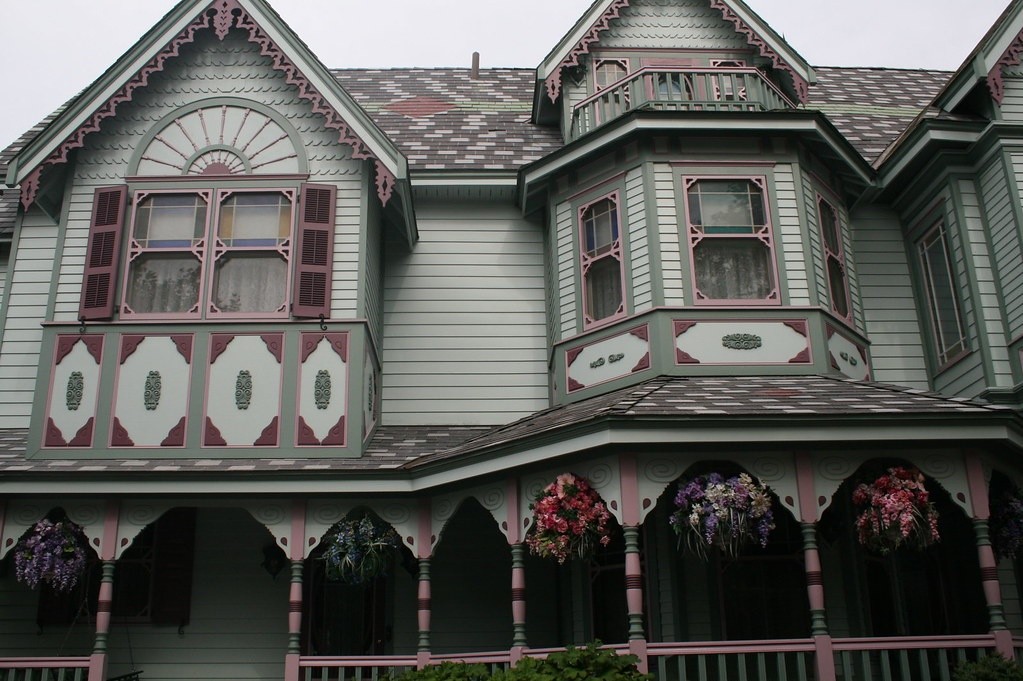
260 543 287 581
401 545 419 579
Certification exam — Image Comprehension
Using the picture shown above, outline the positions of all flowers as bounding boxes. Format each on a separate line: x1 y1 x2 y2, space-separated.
987 484 1023 561
670 472 772 564
316 513 400 586
12 522 93 592
524 476 610 563
848 465 947 554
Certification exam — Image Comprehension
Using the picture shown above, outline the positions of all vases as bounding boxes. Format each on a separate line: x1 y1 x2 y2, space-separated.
690 515 749 546
24 570 90 602
538 528 599 560
867 516 921 546
329 542 391 582
